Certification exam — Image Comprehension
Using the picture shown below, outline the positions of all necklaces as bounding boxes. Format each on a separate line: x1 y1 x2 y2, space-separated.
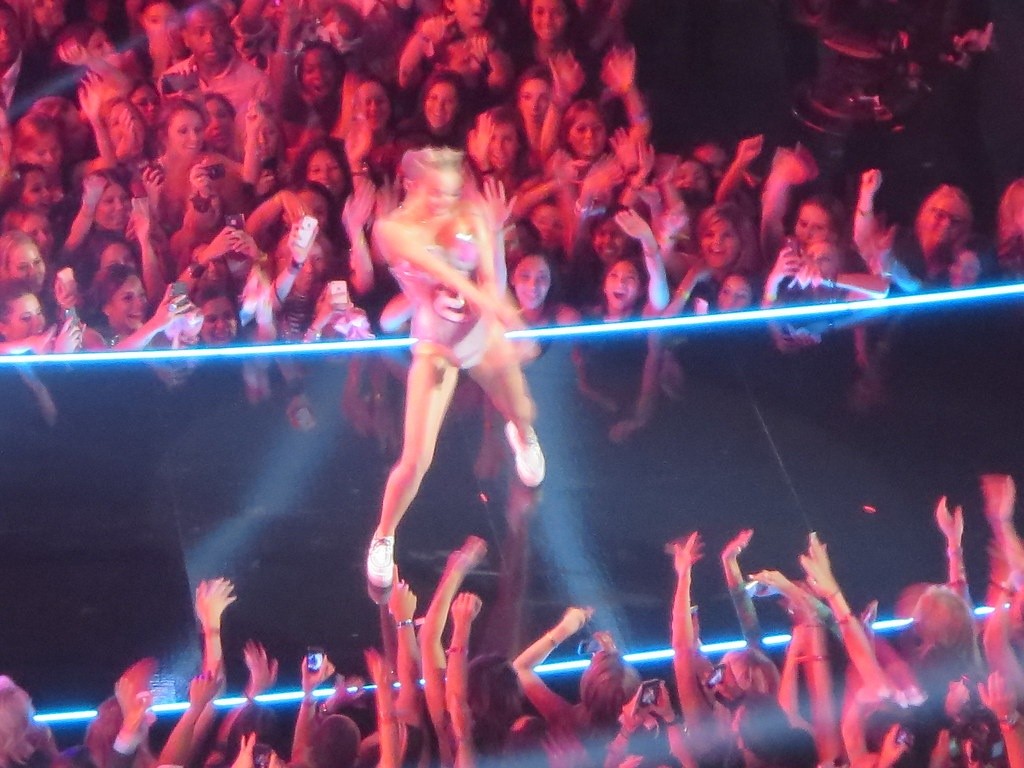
398 204 437 225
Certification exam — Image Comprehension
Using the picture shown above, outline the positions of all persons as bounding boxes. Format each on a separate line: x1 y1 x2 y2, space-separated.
366 144 547 588
0 473 1024 767
0 0 987 356
995 173 1024 281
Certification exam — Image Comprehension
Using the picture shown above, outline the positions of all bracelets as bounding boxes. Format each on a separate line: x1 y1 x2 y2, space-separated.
999 710 1020 727
639 246 664 259
443 645 470 655
947 548 963 558
241 692 259 705
573 198 594 218
287 256 302 274
674 286 692 302
619 727 633 741
825 588 841 600
255 251 269 266
272 45 301 59
396 617 415 628
661 714 683 726
852 204 877 217
546 632 560 649
319 702 331 716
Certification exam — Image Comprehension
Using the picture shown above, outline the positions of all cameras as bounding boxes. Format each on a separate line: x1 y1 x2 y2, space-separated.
203 163 225 180
706 664 726 689
896 727 916 753
745 580 767 598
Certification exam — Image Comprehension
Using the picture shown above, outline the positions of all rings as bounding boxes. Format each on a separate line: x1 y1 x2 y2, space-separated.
166 302 177 311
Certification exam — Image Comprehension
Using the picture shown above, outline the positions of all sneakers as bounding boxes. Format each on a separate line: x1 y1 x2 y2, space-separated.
505 419 545 487
366 536 394 588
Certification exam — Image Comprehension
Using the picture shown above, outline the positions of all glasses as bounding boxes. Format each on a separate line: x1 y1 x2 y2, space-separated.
927 206 967 224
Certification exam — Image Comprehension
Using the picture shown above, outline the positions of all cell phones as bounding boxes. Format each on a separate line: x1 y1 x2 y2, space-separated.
226 213 243 232
689 606 699 616
173 281 187 308
294 215 319 250
259 156 279 171
139 161 164 184
63 307 79 327
161 69 199 95
783 236 799 258
253 744 272 768
331 280 349 304
306 646 324 672
638 679 660 708
578 641 602 654
